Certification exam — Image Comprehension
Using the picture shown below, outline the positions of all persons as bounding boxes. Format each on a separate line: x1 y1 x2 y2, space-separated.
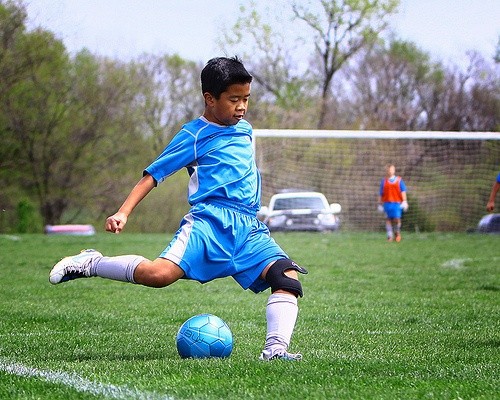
376 163 410 241
48 57 309 365
486 174 500 212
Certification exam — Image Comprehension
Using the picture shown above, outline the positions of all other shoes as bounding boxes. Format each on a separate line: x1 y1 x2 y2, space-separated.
386 237 393 242
394 233 401 242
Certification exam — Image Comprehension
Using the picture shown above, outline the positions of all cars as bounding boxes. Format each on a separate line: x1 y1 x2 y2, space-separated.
465 213 500 234
259 191 342 233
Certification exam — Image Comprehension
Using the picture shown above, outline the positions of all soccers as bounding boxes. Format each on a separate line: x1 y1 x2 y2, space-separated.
176 313 234 359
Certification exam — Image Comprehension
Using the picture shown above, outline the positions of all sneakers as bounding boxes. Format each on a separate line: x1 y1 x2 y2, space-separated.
258 349 303 362
49 248 103 284
